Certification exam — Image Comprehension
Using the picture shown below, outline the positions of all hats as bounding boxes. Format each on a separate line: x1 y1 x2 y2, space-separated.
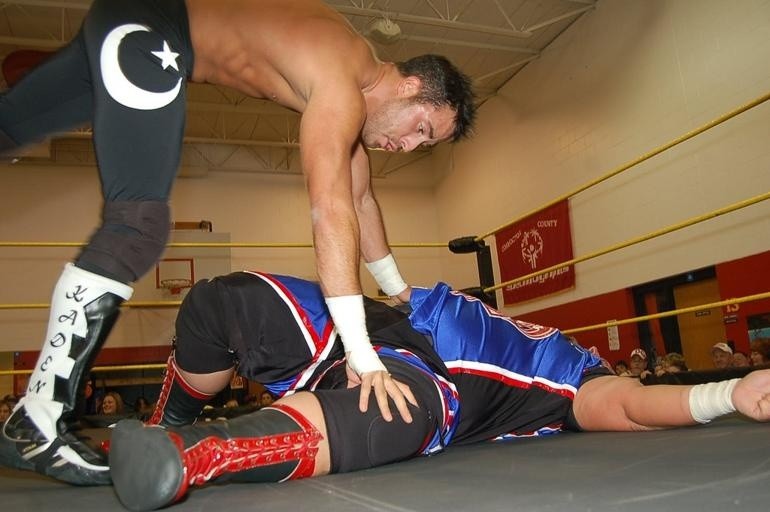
711 343 732 355
630 348 647 360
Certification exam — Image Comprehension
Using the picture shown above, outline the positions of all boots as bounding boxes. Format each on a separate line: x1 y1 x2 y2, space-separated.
101 351 324 511
0 261 134 486
